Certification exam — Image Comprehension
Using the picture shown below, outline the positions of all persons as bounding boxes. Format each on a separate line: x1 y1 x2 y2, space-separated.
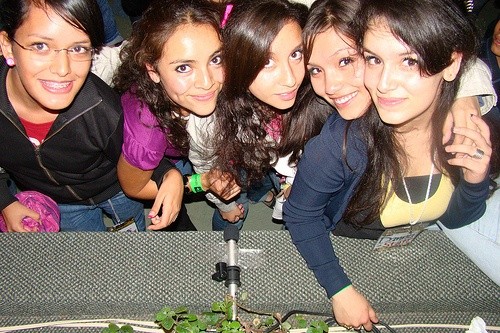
278 0 495 333
209 1 316 233
464 0 500 133
0 0 149 235
94 1 124 49
113 0 252 231
266 0 499 222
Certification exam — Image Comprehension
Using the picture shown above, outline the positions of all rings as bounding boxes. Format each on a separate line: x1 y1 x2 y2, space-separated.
472 149 485 160
354 326 361 330
343 325 352 330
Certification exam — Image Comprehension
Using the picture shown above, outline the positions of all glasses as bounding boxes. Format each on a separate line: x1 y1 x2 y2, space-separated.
8 36 98 59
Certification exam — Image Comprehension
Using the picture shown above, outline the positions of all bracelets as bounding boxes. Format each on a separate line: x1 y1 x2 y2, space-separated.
188 175 205 194
